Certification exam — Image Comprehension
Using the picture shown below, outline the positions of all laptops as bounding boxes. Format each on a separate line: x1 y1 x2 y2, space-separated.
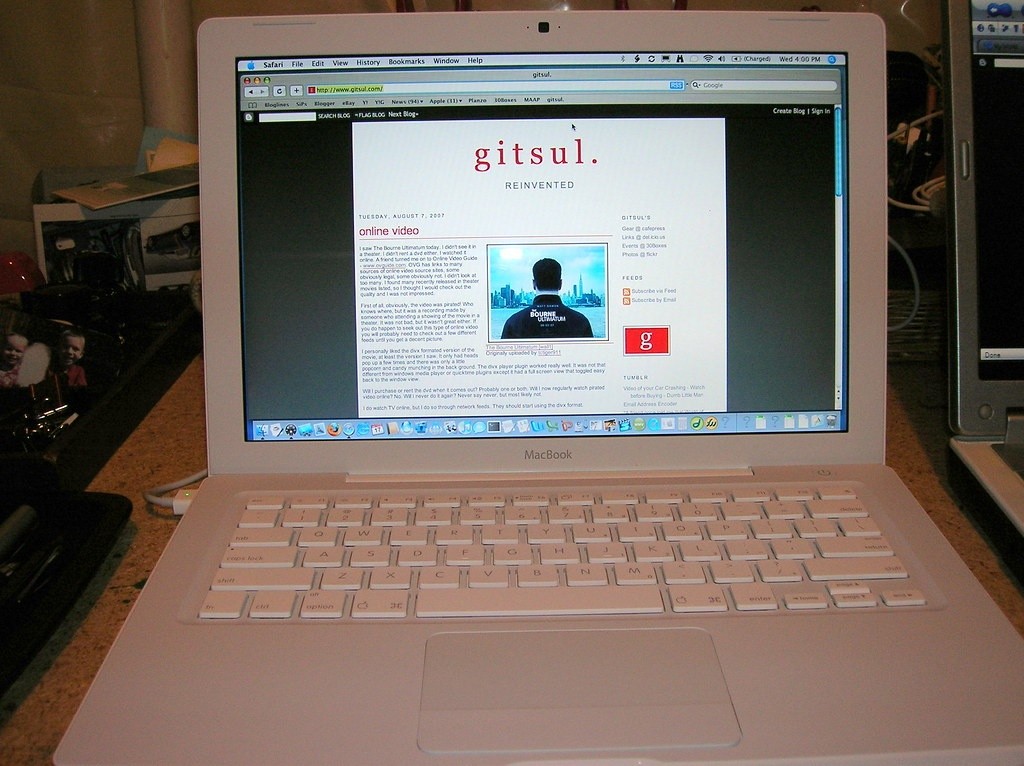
938 0 1024 581
55 10 1023 766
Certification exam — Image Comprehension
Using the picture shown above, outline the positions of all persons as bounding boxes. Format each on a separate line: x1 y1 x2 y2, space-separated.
52 331 88 389
499 258 594 339
0 333 28 389
